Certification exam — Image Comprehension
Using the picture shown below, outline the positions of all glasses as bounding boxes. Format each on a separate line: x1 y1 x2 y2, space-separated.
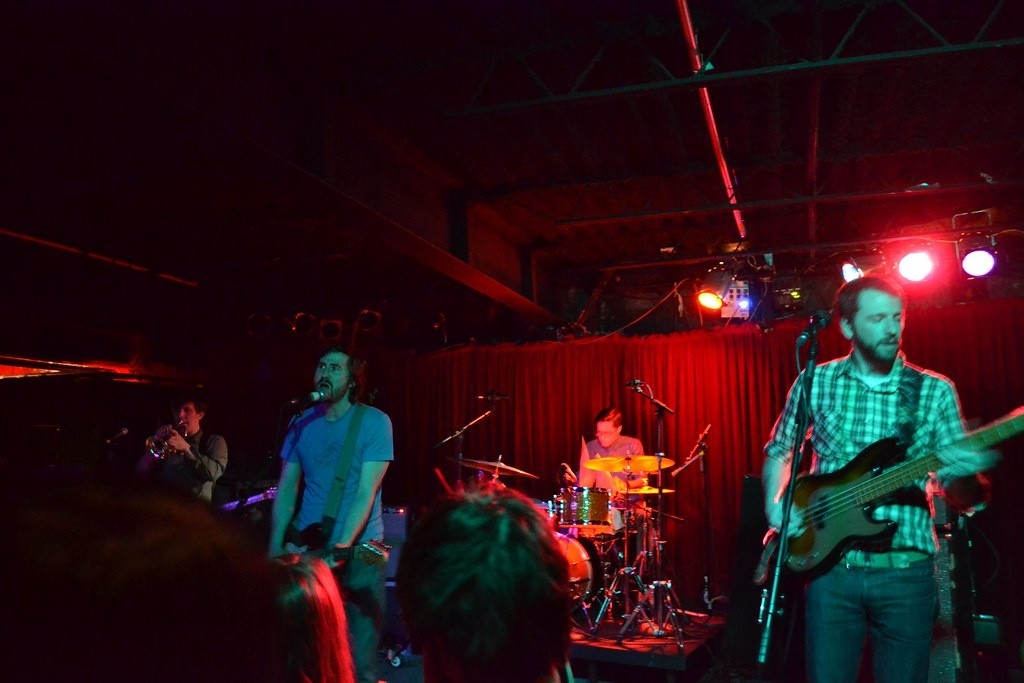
595 430 618 437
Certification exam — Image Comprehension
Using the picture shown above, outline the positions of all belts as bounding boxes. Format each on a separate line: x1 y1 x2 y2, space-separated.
842 550 929 569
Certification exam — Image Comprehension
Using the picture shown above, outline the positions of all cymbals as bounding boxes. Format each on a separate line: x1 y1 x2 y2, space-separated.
584 455 676 473
458 457 540 478
618 485 676 494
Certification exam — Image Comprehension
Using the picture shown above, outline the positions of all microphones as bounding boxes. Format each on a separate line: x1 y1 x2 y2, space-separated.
283 392 325 409
477 394 511 400
107 427 128 443
671 452 705 476
795 310 833 347
625 381 646 386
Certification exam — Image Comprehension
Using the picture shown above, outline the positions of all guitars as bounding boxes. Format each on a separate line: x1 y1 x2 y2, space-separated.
306 539 392 569
779 402 1023 585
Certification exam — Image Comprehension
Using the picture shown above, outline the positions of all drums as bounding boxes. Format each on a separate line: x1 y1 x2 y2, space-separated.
554 531 598 611
555 486 611 526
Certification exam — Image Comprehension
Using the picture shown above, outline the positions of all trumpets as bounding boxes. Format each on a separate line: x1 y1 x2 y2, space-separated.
145 418 189 460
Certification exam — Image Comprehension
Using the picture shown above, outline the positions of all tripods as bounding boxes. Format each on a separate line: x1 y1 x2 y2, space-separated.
584 385 694 655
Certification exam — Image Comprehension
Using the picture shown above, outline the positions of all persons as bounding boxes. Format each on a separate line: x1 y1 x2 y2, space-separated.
0 494 295 683
578 409 662 583
136 398 229 501
762 278 990 683
242 555 360 682
397 493 585 683
269 347 393 683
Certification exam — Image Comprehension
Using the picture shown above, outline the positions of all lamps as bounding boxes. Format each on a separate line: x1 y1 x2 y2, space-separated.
950 221 997 277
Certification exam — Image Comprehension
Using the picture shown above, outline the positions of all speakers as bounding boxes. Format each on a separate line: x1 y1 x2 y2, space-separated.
381 507 412 587
719 475 806 683
